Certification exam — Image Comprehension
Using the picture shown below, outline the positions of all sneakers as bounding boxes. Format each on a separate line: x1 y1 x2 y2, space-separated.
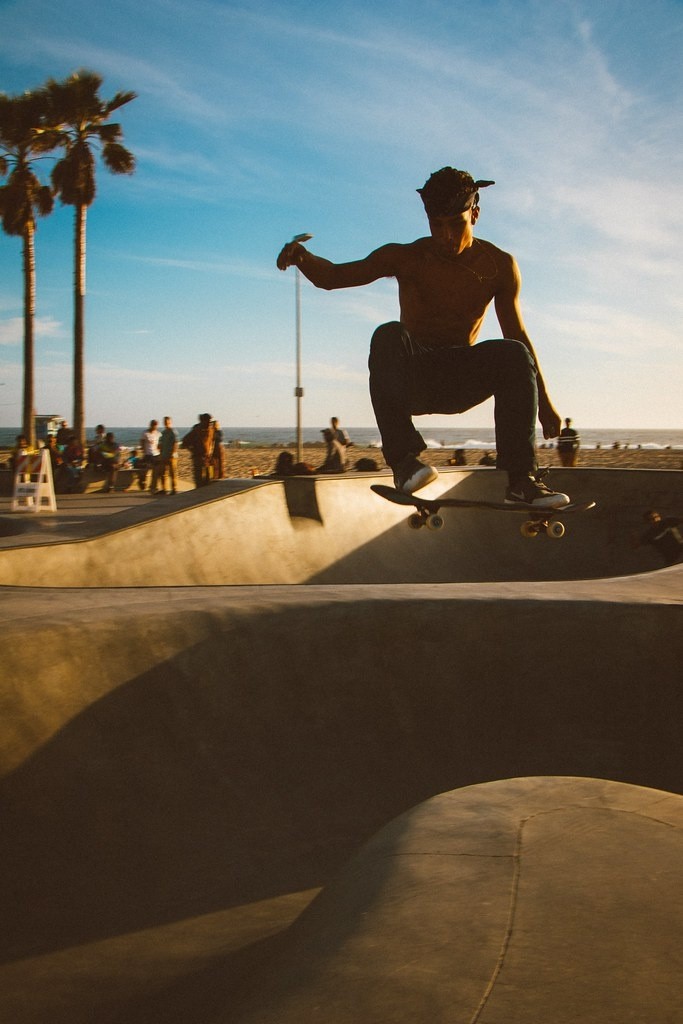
393 459 439 493
503 469 571 509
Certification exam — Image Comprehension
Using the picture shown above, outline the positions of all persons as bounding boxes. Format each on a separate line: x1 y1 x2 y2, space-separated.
12 412 224 496
624 510 683 567
278 416 581 474
272 167 571 511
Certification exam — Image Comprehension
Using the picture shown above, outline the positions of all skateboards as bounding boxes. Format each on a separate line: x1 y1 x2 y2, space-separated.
370 484 596 539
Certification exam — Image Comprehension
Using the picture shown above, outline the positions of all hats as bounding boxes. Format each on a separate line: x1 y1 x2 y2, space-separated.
97 425 103 430
104 433 113 439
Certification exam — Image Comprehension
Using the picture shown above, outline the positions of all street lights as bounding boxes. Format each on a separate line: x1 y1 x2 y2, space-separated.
293 233 315 463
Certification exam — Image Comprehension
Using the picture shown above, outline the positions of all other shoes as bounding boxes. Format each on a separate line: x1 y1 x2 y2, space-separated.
170 490 177 495
156 490 168 494
